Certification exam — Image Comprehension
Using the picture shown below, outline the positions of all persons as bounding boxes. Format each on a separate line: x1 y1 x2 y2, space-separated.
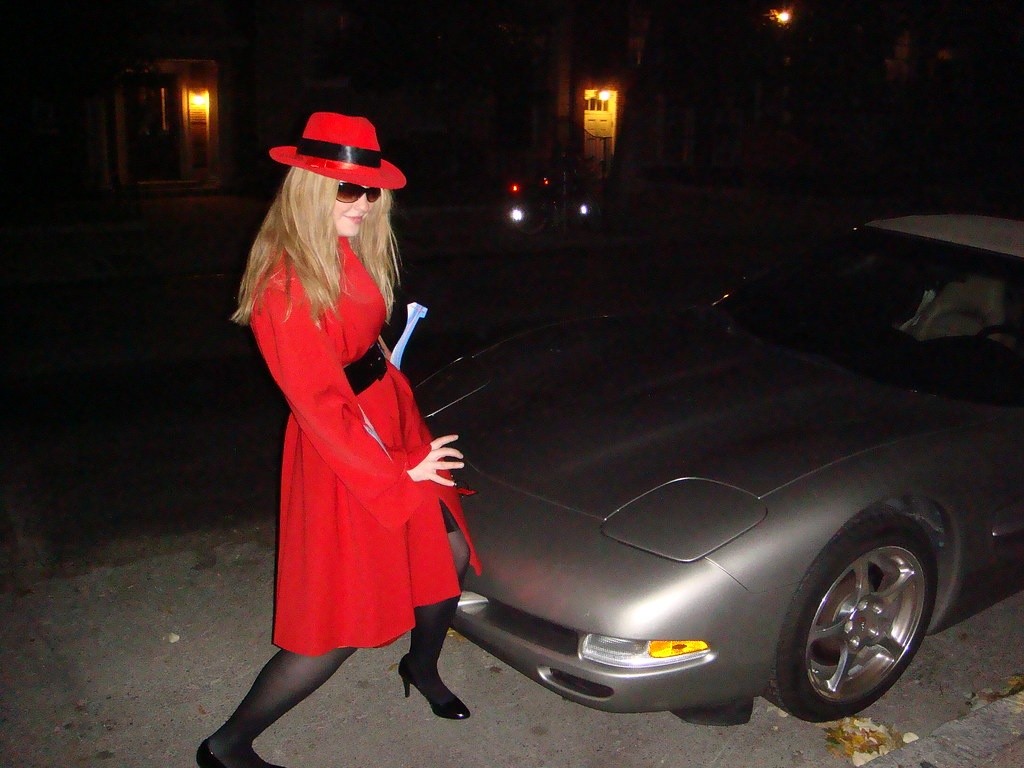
196 113 484 768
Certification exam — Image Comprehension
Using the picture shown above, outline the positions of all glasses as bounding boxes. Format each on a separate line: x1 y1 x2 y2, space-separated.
336 183 381 204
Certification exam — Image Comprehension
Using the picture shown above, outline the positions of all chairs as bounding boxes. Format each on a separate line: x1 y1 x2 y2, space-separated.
911 272 1008 342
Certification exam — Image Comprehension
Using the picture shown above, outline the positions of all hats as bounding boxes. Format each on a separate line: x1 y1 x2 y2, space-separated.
269 112 407 190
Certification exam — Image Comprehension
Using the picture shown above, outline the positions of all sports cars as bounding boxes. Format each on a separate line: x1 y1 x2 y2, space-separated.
409 208 1023 728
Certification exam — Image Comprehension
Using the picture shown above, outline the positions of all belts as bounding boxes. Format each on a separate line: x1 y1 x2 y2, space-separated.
344 339 388 395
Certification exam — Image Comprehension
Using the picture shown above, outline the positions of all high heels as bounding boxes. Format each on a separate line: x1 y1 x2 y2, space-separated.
195 739 284 768
398 653 470 719
